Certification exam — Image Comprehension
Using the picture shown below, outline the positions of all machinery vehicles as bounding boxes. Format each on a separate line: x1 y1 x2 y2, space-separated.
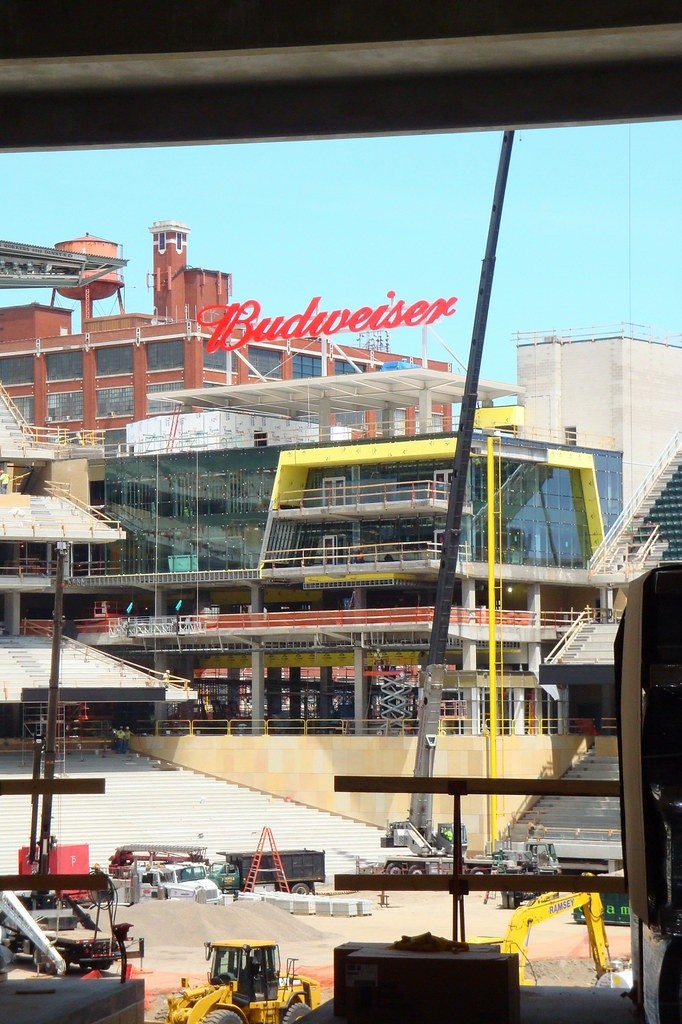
163 936 321 1024
451 870 623 989
345 121 564 910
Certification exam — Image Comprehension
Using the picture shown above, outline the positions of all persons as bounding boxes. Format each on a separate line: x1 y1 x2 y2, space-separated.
0 470 11 494
354 550 366 564
444 827 453 842
115 725 134 754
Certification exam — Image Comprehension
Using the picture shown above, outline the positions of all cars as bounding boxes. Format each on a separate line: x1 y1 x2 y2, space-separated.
0 887 122 977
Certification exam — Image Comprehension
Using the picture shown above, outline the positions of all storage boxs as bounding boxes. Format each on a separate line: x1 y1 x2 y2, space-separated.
220 891 373 917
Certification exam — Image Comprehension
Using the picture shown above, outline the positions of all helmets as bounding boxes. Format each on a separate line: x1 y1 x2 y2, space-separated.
125 726 129 730
120 726 123 728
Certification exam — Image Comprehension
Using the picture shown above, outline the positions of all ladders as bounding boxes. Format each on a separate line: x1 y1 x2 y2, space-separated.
243 826 290 893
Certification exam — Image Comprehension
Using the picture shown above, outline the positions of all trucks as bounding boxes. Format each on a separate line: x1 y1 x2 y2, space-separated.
202 847 326 896
104 841 226 907
